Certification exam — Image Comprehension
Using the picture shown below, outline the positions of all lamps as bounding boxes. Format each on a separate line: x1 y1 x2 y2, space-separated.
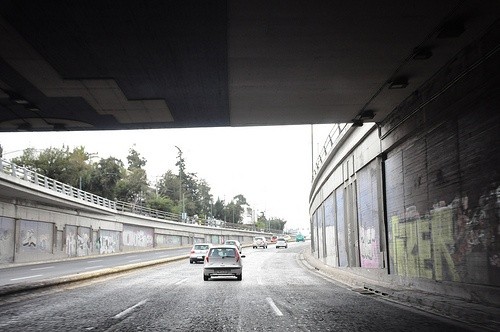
388 77 409 89
8 94 39 112
412 47 433 60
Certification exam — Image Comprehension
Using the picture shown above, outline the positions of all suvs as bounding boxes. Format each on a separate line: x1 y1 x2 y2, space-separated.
252 236 268 249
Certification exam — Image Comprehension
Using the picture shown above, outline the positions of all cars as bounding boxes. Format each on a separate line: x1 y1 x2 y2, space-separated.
202 244 246 281
271 235 277 242
189 243 214 264
275 238 288 248
224 240 243 254
295 234 305 242
